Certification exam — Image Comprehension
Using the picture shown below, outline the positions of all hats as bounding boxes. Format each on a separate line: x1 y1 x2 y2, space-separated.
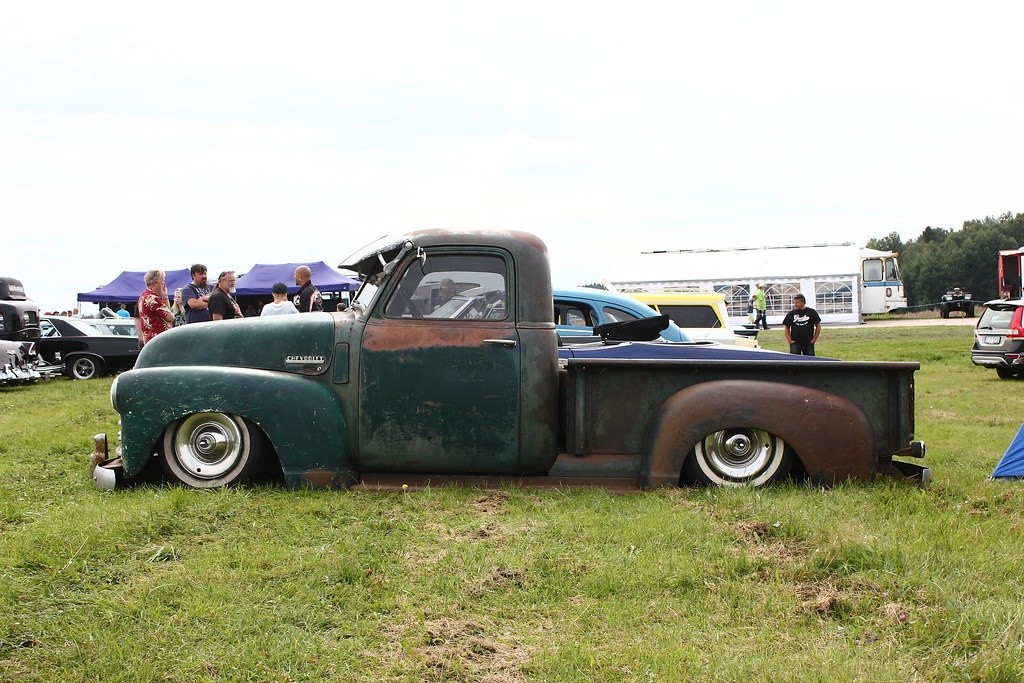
758 283 765 287
272 282 287 294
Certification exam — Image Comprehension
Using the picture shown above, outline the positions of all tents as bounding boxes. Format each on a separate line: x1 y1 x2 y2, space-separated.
76 269 196 319
227 261 364 317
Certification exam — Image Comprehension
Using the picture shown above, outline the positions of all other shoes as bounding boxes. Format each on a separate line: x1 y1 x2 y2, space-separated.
764 327 771 330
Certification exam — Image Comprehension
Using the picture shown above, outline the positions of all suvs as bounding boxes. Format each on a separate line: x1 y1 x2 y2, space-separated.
970 298 1024 380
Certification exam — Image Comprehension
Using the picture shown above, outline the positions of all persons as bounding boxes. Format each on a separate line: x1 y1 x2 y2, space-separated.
756 282 771 330
260 282 300 316
142 273 175 345
294 266 323 312
44 308 80 319
336 303 345 312
134 296 145 350
181 264 214 324
208 270 244 320
782 294 821 356
434 279 457 310
171 288 187 326
115 304 130 317
746 295 758 324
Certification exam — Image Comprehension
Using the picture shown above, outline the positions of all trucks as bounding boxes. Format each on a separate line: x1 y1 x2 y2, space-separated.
0 276 41 383
997 246 1024 300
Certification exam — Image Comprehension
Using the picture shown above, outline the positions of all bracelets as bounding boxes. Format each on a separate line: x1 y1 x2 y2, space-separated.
164 297 168 300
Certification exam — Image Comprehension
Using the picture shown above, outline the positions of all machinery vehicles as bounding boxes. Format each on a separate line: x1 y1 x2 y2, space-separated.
941 286 974 318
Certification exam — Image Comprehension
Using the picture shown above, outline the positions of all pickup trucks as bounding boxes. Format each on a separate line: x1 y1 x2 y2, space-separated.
87 227 932 492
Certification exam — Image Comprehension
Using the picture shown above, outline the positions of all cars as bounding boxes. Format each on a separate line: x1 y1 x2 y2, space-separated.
24 317 145 380
423 285 761 349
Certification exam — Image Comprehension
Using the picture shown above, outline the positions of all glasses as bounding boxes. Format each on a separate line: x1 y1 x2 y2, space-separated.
222 271 235 277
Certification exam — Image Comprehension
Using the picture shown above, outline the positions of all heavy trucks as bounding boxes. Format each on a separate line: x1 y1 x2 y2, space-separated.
861 247 908 316
